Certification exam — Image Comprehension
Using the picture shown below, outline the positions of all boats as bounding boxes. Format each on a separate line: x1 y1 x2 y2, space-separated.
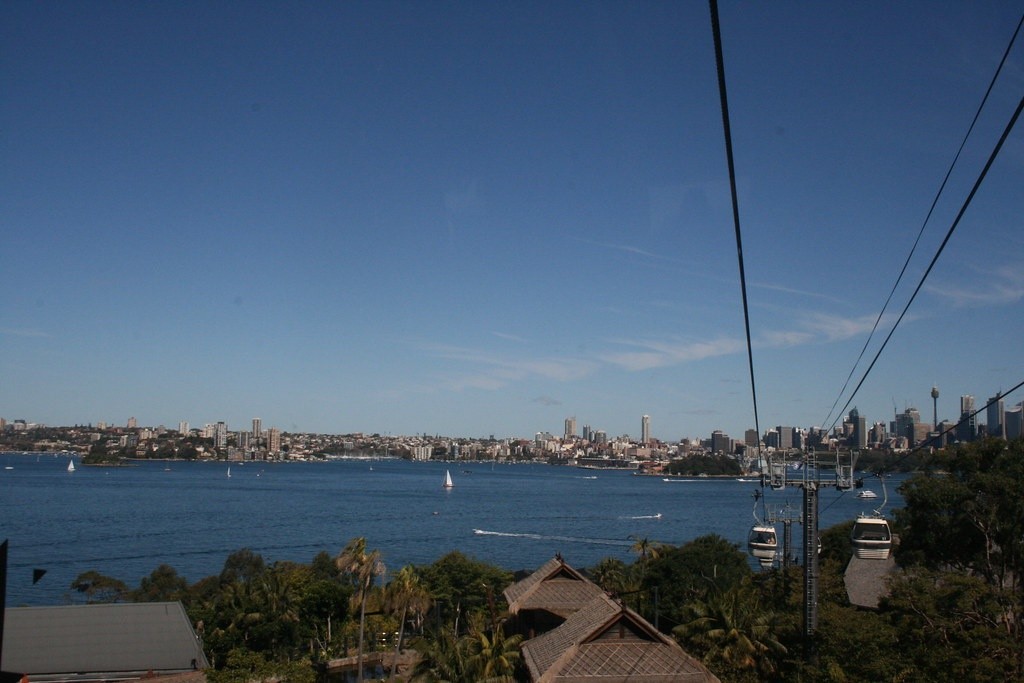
855 490 878 499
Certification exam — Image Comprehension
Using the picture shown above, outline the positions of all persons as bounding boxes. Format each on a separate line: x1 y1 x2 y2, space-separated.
837 476 849 487
768 532 774 544
753 533 766 543
775 476 783 484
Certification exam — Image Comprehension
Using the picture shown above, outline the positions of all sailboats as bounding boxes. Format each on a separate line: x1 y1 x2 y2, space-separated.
442 470 455 487
